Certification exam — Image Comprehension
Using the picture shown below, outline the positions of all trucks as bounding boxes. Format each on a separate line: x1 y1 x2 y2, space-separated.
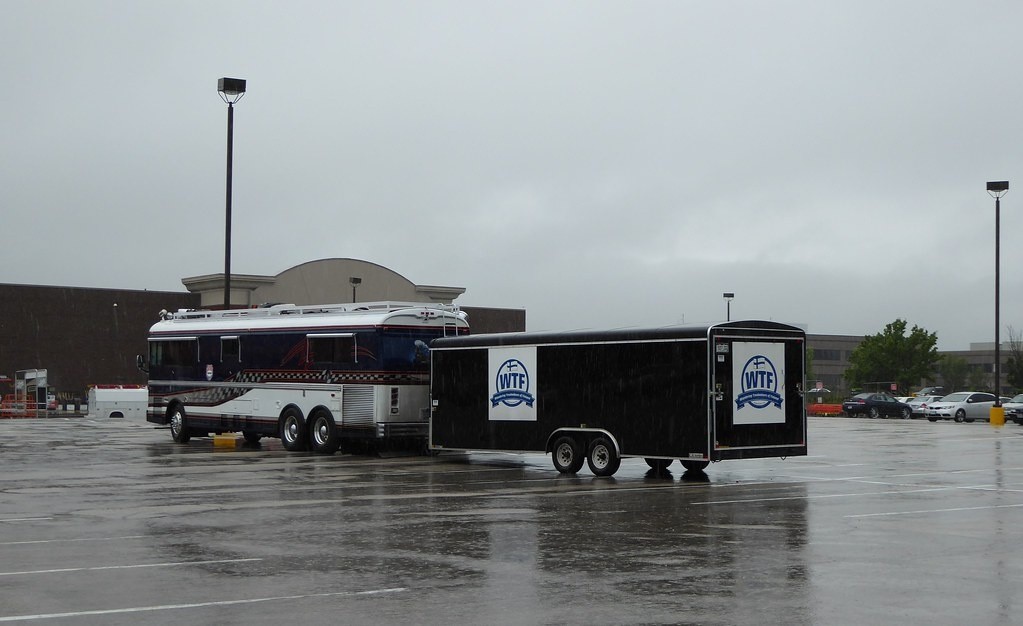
135 301 807 476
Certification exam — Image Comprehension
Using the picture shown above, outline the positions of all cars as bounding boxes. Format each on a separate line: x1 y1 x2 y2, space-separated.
842 386 1023 425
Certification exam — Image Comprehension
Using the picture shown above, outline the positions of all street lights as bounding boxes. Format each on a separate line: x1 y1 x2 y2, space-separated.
987 181 1009 426
218 78 246 310
723 292 734 321
349 277 361 303
